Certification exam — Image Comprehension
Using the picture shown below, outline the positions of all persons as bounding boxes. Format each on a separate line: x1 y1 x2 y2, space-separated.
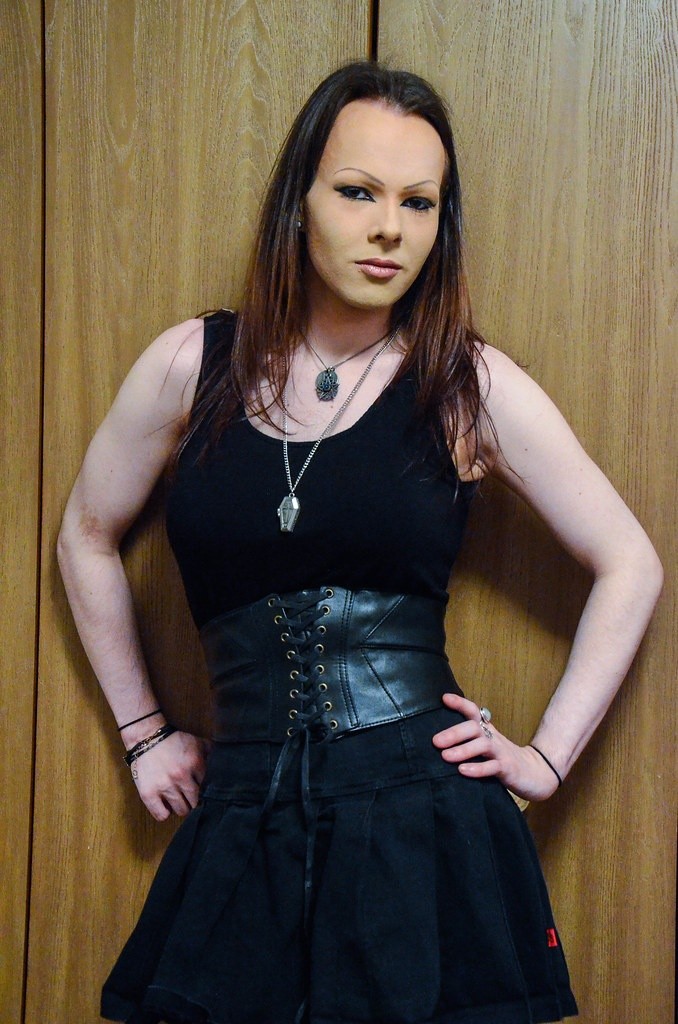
58 64 663 1023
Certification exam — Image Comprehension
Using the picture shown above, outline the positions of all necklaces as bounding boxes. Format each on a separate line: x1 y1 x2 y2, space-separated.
289 329 393 401
275 326 402 534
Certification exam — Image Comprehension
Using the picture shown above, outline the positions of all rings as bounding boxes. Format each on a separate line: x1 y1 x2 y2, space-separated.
482 727 491 736
479 707 491 722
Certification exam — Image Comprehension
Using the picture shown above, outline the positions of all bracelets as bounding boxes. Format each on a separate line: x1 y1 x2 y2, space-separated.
119 709 162 731
122 723 179 780
529 744 562 788
